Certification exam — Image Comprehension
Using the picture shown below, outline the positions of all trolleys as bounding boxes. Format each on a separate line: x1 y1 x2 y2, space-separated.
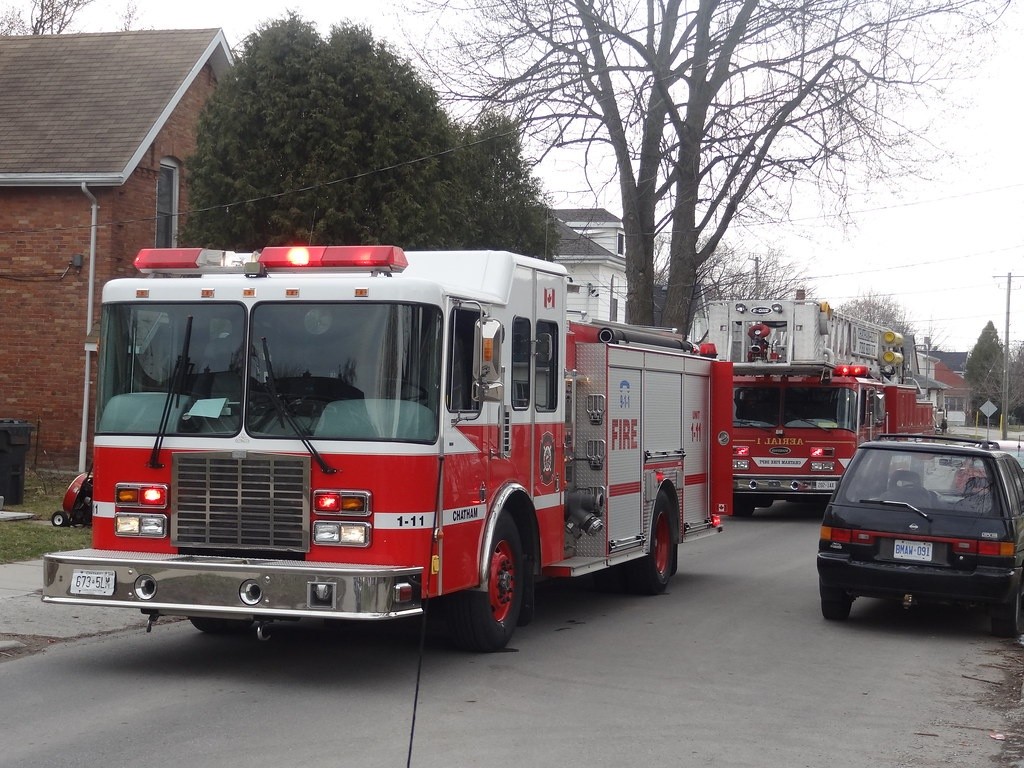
52 460 93 527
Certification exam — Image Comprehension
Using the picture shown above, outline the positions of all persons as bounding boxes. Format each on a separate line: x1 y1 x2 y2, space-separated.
212 334 287 410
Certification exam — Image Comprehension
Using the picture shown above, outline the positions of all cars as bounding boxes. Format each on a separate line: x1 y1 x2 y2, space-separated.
816 433 1024 638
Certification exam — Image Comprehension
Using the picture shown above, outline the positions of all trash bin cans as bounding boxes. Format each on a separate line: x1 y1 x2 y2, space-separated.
0 417 36 505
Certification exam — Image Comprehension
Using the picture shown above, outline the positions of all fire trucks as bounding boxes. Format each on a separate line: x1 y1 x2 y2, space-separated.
39 245 734 657
731 360 935 515
